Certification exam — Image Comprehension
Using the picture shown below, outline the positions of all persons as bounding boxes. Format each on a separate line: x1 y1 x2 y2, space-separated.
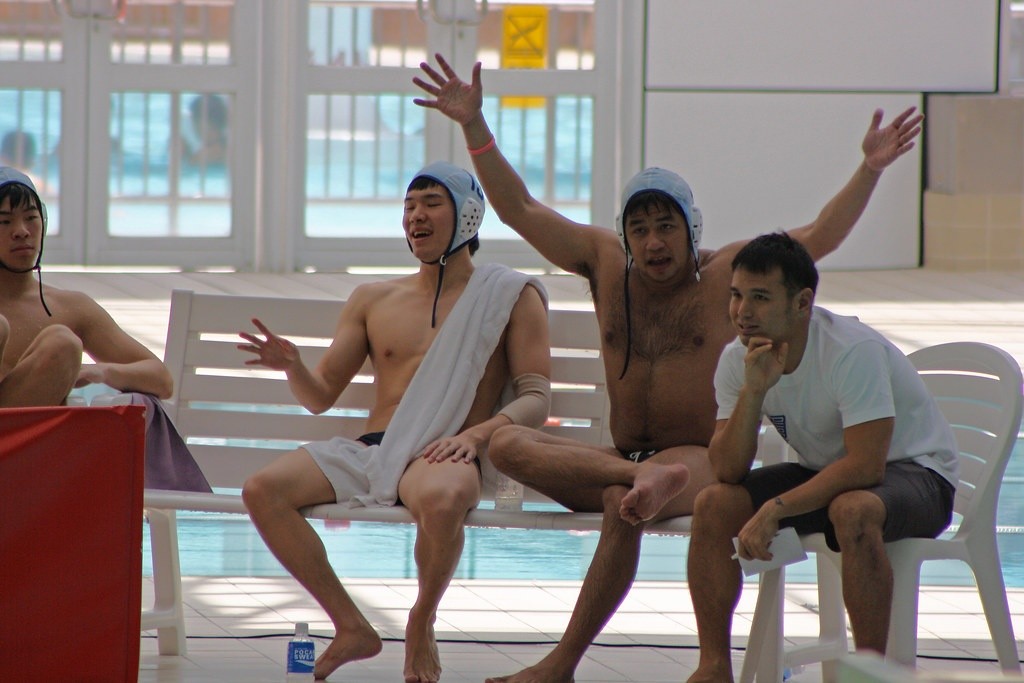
686 229 959 683
413 51 924 683
0 166 174 409
177 94 229 171
0 131 57 205
237 161 551 683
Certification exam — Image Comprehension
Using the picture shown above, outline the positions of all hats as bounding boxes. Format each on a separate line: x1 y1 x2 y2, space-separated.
403 160 485 259
616 166 703 262
0 164 47 246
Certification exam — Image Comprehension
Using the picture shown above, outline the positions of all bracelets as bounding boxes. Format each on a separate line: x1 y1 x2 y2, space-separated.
466 134 496 156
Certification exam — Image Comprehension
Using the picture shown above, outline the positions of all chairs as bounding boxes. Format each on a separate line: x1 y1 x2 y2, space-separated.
732 339 1023 683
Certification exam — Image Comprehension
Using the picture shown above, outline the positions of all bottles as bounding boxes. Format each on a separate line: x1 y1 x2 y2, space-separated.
494 471 524 512
285 622 316 683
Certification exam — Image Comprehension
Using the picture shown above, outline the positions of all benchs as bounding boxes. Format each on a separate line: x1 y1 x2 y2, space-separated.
89 283 850 683
